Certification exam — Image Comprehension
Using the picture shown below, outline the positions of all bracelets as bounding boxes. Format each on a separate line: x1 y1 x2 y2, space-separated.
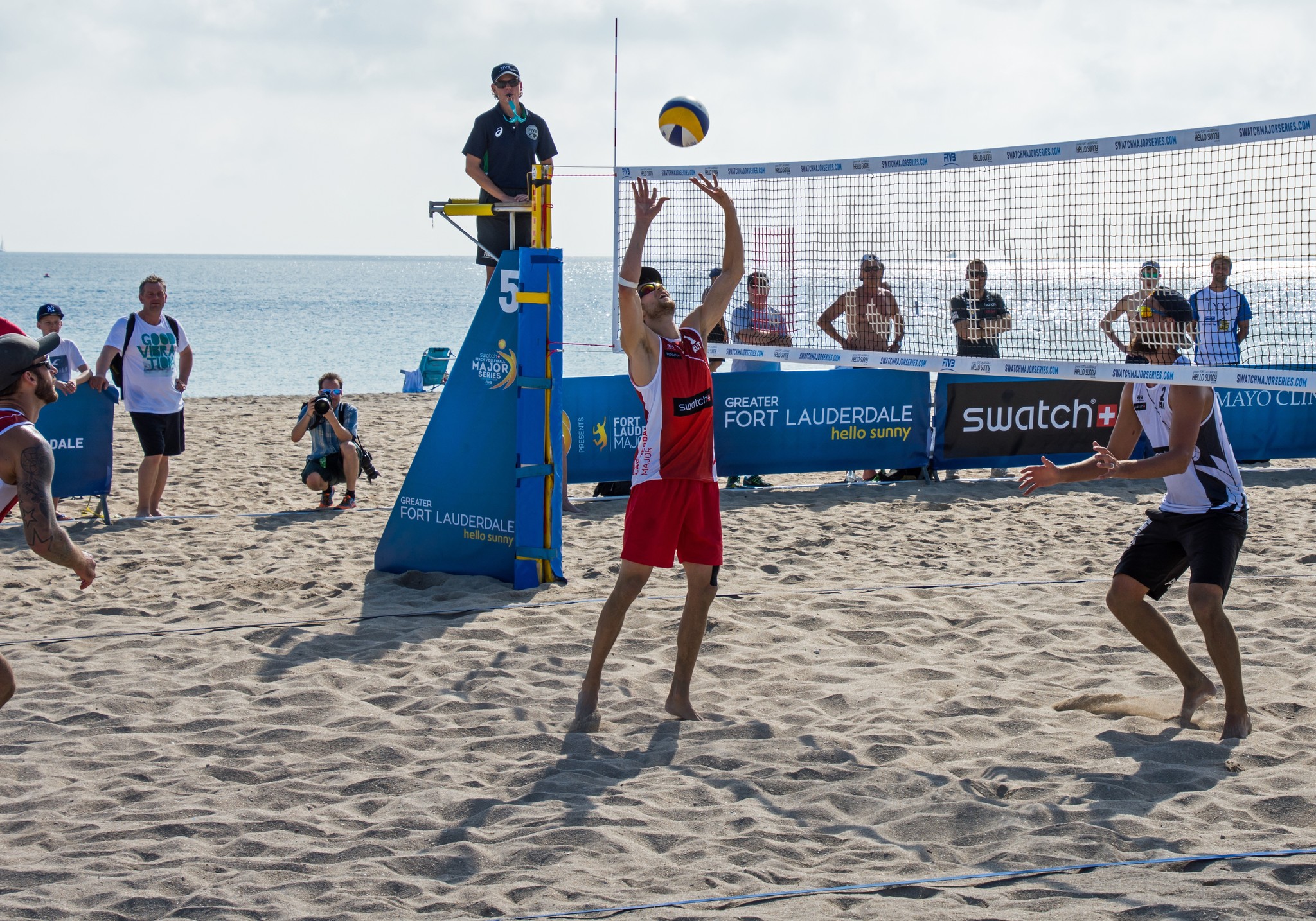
306 411 313 417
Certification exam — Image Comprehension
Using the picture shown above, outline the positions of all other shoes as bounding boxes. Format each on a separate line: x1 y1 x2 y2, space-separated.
333 493 357 511
319 485 335 508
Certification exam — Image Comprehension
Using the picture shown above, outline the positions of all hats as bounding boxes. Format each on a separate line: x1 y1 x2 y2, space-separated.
1141 259 1160 272
36 303 64 321
0 332 61 389
747 271 769 284
860 254 881 281
1151 286 1193 349
491 63 520 83
637 266 663 286
709 268 722 278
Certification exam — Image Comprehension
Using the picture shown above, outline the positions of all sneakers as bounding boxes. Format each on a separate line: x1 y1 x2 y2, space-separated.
743 475 774 487
991 468 1015 478
726 475 740 488
945 469 961 479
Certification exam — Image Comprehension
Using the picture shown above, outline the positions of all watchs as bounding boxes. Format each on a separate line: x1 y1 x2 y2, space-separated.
893 340 902 347
976 320 980 328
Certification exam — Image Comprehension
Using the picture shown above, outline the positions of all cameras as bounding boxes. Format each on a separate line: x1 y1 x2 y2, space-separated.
361 450 383 484
311 393 332 415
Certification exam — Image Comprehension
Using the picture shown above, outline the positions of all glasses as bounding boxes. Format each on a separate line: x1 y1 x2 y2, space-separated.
1136 305 1170 318
638 283 656 301
495 79 519 88
1142 273 1160 278
12 354 51 375
750 283 769 289
320 389 342 395
970 271 987 277
864 266 881 272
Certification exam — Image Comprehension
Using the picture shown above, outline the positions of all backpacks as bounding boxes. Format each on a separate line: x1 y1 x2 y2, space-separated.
108 313 180 387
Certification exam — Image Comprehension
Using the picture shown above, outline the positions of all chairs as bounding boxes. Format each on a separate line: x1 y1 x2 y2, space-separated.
35 381 120 526
400 347 457 393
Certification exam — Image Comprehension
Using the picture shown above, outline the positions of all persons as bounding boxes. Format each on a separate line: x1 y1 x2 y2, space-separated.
0 275 192 706
291 372 363 510
574 173 746 722
461 63 559 293
1016 285 1254 741
698 255 1258 490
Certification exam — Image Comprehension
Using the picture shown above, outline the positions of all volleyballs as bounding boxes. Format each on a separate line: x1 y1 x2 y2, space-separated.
658 94 709 147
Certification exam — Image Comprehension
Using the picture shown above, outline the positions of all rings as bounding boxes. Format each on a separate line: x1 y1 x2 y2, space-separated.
1109 463 1115 469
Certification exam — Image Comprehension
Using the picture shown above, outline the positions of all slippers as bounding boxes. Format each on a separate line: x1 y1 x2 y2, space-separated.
844 478 857 482
863 473 883 482
55 514 76 521
